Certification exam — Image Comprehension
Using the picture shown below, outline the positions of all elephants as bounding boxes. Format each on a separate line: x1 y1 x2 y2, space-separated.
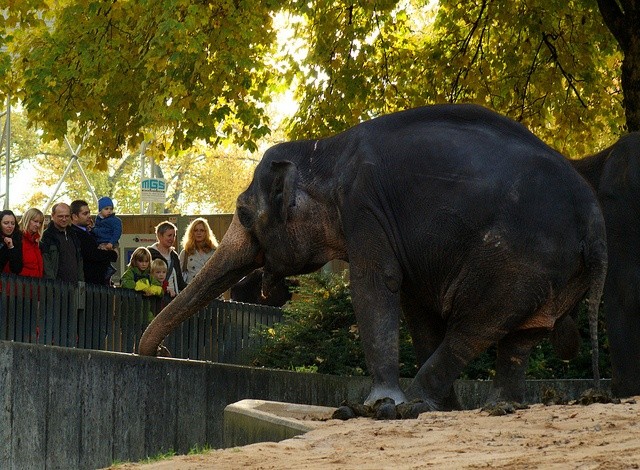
549 131 640 398
138 103 608 419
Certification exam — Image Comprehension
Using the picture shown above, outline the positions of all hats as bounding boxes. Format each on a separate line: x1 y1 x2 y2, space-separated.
98 197 113 210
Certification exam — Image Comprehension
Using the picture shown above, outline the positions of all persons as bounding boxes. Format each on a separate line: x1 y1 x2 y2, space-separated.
119 246 165 354
39 202 84 348
149 257 169 298
178 216 225 361
0 207 45 344
0 207 24 274
69 199 119 350
92 195 123 279
146 219 189 297
